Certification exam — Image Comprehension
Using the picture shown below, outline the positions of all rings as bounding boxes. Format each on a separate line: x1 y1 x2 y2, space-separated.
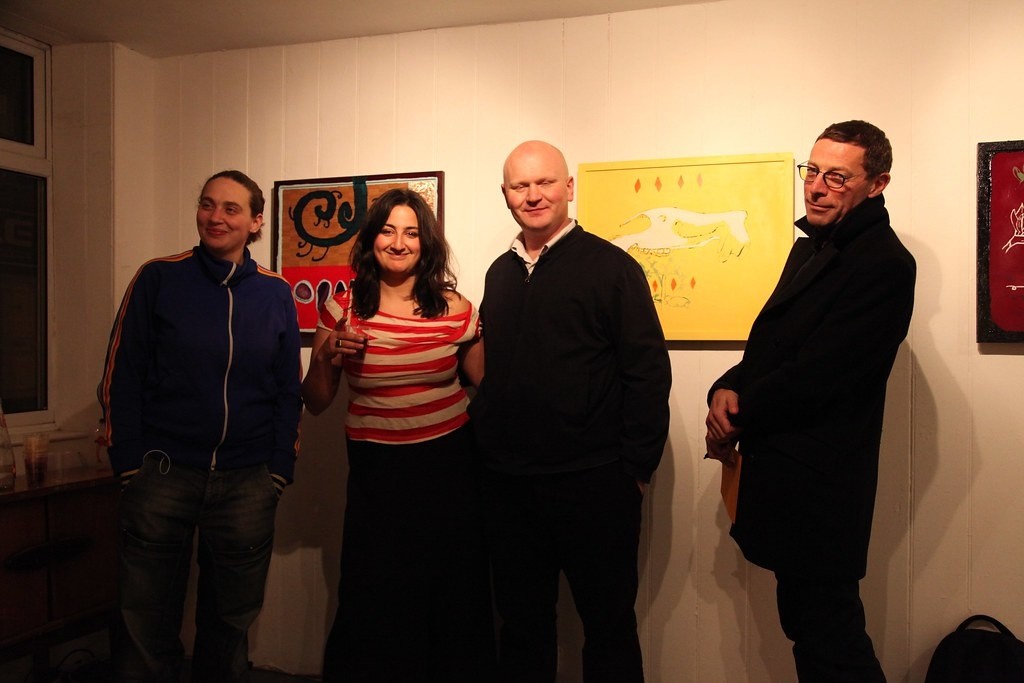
336 340 341 347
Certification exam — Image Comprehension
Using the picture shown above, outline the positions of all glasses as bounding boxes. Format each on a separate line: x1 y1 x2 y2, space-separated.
798 164 872 189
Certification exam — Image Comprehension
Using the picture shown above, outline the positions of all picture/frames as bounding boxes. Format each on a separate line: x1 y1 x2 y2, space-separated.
975 140 1024 343
577 152 795 341
271 170 445 343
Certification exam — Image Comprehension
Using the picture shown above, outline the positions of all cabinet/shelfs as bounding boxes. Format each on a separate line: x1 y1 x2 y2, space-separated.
0 465 122 683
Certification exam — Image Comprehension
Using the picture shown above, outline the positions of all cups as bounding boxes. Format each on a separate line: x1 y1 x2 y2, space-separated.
24 433 49 486
344 325 370 362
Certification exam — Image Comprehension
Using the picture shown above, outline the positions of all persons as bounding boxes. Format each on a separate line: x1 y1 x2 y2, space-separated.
478 140 672 683
97 171 302 683
301 191 497 683
705 119 916 683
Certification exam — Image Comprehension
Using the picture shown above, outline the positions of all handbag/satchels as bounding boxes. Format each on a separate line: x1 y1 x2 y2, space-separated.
926 614 1024 683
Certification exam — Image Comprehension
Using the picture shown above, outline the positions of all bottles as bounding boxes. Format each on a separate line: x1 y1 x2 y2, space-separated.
91 419 109 470
0 398 16 488
